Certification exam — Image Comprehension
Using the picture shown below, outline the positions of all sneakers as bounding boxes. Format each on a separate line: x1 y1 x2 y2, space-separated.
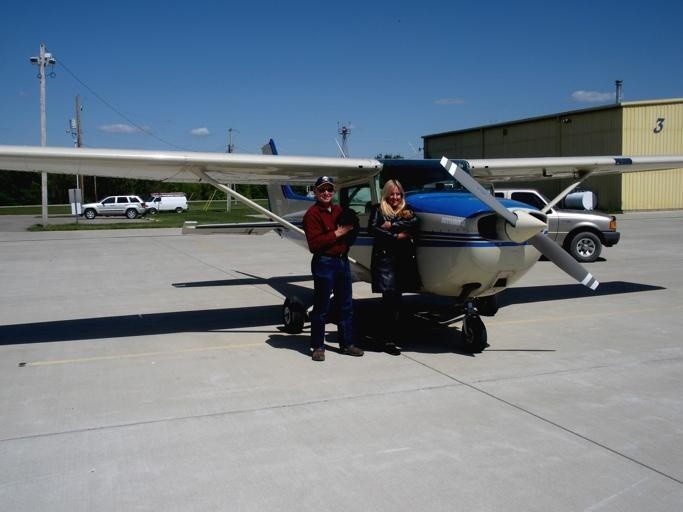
339 345 364 356
312 346 326 361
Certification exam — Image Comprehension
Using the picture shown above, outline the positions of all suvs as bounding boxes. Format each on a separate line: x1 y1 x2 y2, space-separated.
486 188 620 265
80 193 148 220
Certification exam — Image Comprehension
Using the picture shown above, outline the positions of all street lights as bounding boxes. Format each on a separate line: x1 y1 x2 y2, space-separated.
28 43 60 229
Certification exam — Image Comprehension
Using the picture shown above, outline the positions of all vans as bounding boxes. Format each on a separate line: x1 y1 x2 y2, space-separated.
143 192 188 214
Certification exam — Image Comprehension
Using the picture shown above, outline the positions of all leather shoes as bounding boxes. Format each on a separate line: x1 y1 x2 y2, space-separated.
383 343 401 355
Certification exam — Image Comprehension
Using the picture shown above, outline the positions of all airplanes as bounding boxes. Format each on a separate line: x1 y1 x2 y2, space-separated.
0 139 682 354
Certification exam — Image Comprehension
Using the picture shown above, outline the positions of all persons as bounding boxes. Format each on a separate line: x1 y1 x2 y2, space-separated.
303 175 364 361
367 179 421 355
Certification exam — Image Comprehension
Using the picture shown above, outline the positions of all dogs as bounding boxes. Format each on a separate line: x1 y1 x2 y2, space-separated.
397 210 413 239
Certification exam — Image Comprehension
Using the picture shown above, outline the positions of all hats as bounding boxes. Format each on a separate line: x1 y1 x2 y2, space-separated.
314 176 336 189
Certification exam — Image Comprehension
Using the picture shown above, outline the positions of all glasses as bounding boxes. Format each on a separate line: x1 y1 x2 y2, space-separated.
319 188 334 192
